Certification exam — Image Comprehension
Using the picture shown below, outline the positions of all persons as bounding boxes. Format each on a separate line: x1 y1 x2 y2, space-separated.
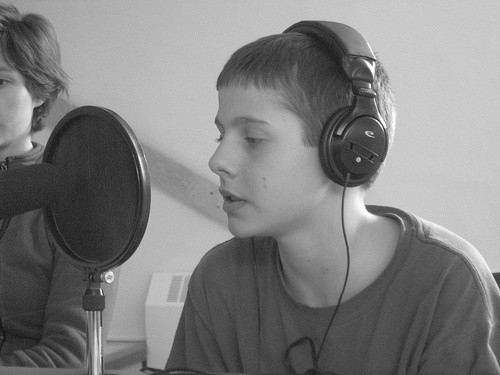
0 4 89 369
163 20 500 375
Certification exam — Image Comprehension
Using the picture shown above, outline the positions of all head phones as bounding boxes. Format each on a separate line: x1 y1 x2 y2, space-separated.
281 20 389 187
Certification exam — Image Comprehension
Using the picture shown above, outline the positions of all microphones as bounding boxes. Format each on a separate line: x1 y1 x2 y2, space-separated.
0 162 60 221
